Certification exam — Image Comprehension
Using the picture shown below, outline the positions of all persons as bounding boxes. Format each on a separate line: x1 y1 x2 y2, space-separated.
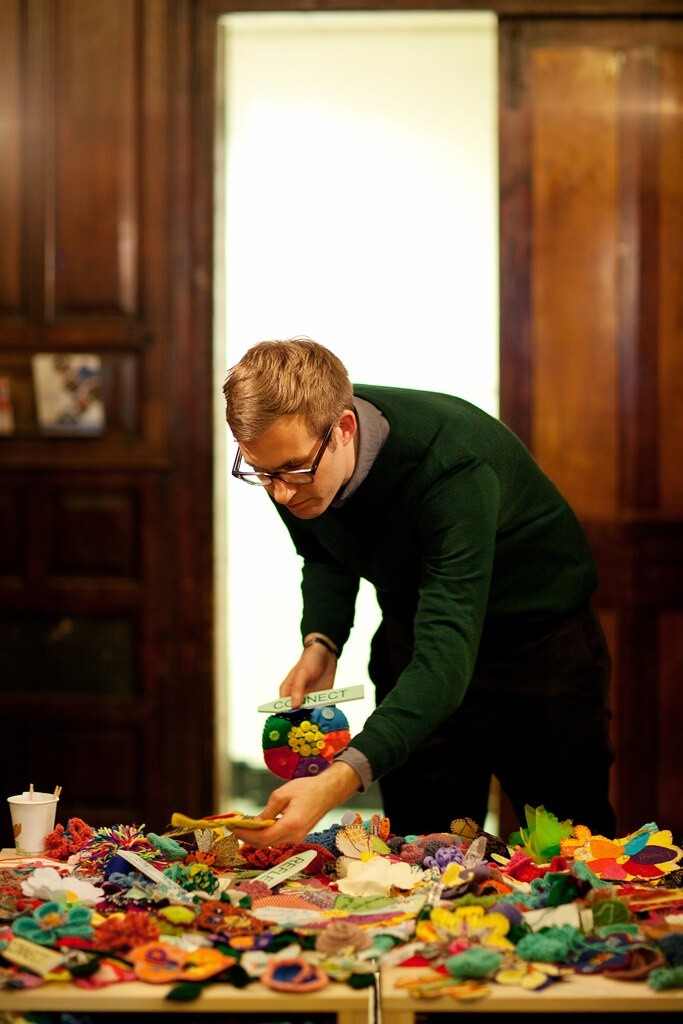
223 341 617 842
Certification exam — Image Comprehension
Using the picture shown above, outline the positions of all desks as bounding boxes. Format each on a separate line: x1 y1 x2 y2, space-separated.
1 827 683 1024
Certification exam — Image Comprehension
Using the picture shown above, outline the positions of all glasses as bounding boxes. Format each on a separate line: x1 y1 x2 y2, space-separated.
232 417 337 486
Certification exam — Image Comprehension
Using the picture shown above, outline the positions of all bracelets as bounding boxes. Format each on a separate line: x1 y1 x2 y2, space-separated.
305 638 339 655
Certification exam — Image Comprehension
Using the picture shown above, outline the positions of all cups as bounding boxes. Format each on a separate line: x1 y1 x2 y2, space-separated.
7 793 59 852
